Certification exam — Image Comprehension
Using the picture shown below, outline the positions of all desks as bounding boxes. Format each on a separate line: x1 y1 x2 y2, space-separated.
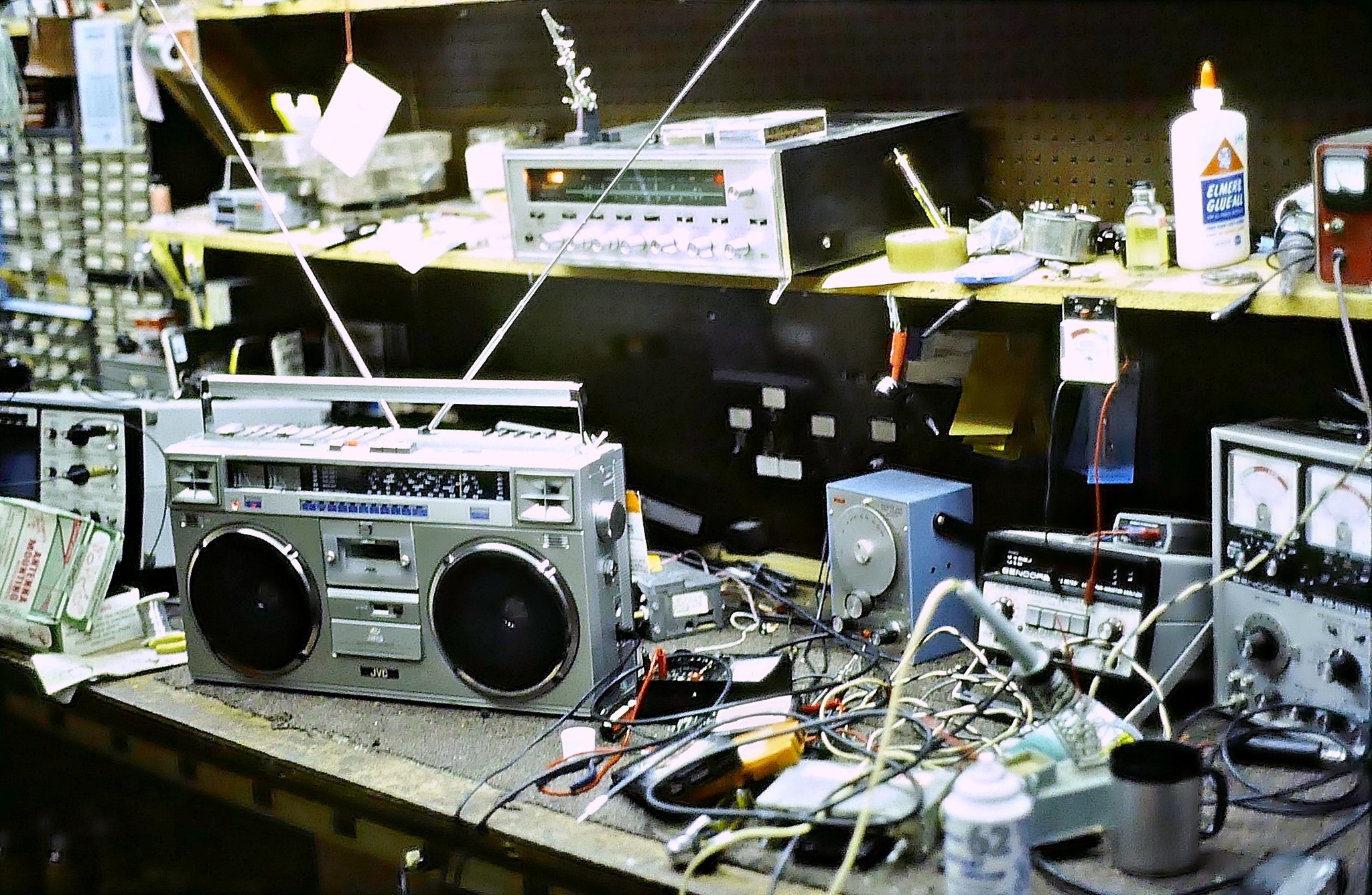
0 566 1372 895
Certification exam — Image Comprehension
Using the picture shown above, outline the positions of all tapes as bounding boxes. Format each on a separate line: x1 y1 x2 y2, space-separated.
1022 210 1102 264
886 225 969 274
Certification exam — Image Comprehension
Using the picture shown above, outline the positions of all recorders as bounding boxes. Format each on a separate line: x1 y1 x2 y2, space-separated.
168 371 632 719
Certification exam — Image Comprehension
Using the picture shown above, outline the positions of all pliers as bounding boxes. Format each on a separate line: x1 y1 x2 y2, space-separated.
147 634 187 653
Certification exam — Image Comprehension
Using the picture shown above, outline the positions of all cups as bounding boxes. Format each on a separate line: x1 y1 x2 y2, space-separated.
561 726 597 760
1106 740 1231 876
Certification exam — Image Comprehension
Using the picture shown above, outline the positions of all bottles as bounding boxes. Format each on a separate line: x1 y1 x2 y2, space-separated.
1168 60 1251 273
942 751 1036 894
1124 180 1169 284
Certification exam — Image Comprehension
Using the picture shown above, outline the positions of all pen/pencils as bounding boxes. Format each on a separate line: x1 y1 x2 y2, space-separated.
892 146 950 234
